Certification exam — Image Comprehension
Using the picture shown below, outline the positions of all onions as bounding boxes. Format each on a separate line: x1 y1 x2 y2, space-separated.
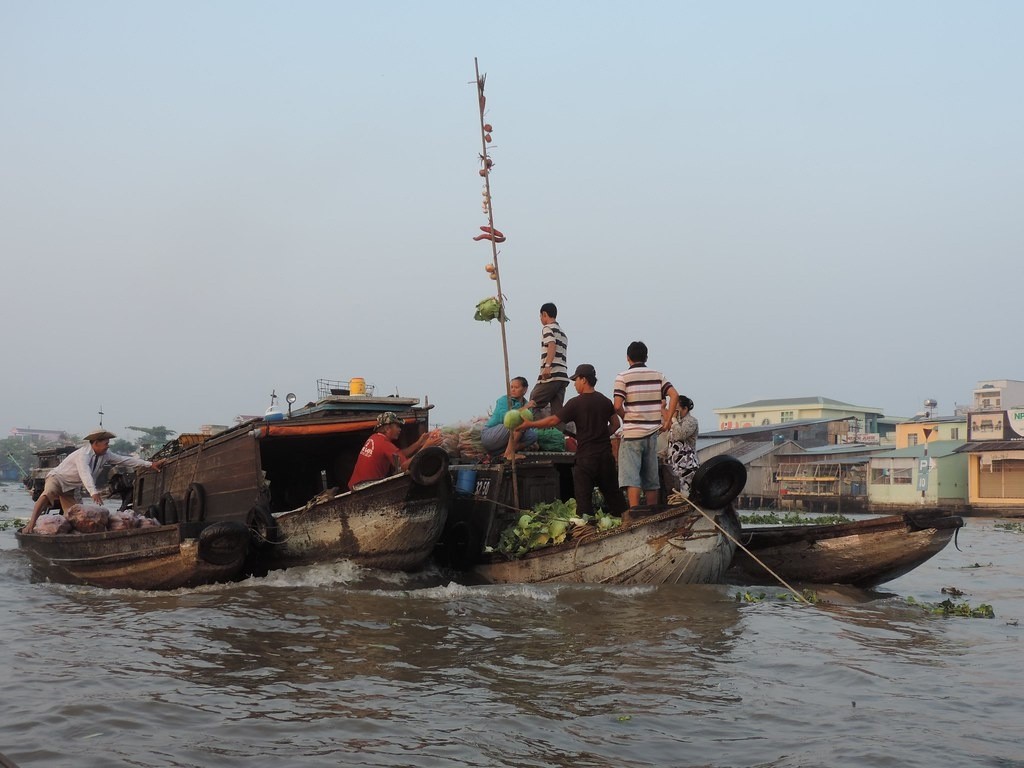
485 264 497 280
479 125 492 176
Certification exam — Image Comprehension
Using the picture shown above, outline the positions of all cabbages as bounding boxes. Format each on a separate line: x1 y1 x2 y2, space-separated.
504 410 533 431
474 297 510 323
487 498 622 555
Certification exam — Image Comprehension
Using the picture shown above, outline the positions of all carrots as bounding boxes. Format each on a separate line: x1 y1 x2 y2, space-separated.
424 427 481 459
473 226 506 243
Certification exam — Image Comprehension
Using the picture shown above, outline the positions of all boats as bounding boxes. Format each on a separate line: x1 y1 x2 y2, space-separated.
13 371 978 591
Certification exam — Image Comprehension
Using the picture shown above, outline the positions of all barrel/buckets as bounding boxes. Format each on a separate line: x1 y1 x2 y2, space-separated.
350 378 365 396
455 468 477 497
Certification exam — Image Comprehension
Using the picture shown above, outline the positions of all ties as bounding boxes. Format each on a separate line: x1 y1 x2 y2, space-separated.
92 455 98 473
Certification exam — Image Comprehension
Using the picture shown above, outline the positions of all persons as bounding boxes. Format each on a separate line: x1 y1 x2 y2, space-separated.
613 341 679 508
480 376 538 460
347 412 445 489
657 394 701 491
514 363 628 517
22 431 166 534
529 303 570 430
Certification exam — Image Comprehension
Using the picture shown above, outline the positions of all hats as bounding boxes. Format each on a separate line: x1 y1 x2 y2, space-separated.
372 411 405 432
569 364 595 381
82 429 116 441
